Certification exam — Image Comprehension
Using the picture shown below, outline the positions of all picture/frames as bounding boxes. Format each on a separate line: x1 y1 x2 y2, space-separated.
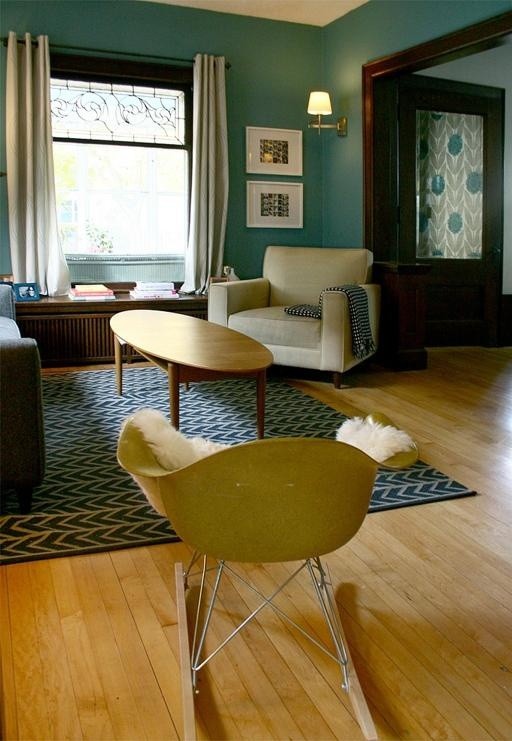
245 127 304 229
14 284 39 301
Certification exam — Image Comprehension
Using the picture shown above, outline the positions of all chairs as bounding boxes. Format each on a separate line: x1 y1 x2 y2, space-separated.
208 246 382 390
116 411 384 741
0 282 45 515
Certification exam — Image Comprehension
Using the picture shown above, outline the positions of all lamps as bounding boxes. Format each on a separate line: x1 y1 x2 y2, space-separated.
308 90 346 138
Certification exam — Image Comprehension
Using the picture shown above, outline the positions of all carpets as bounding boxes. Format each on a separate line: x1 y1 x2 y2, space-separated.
0 372 475 566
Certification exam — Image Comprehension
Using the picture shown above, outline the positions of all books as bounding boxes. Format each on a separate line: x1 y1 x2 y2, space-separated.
67 280 180 301
